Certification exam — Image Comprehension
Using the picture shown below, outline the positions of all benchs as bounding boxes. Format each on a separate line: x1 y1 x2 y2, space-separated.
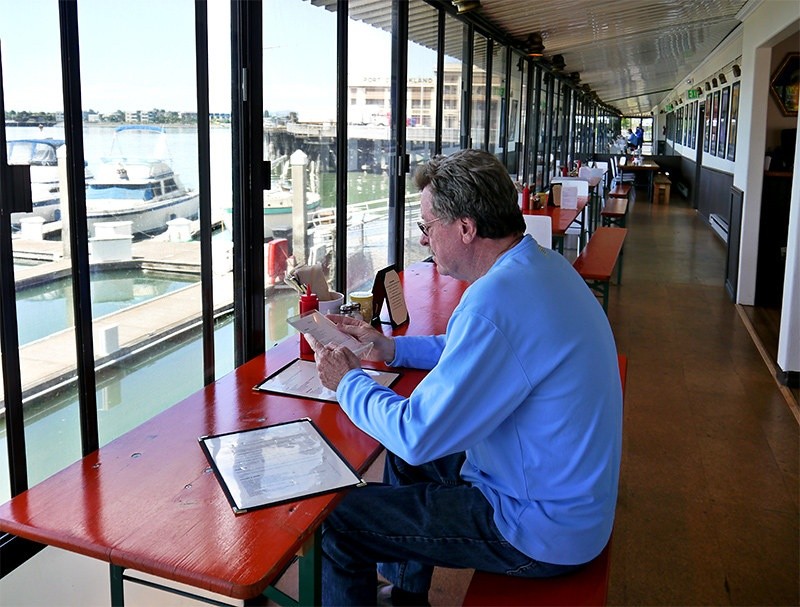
463 351 627 607
608 184 632 212
572 228 630 318
653 175 671 204
602 198 628 229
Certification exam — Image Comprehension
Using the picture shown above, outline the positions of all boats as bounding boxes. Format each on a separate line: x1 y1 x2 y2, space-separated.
6 121 321 283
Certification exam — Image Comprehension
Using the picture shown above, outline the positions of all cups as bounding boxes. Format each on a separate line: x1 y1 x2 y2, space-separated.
765 155 771 172
350 292 374 324
319 292 345 316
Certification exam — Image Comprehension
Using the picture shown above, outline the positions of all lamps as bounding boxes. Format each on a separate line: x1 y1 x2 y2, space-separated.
718 73 727 83
732 65 741 77
712 78 717 88
675 100 678 105
704 82 711 90
679 98 683 104
650 111 653 115
671 104 674 108
442 0 623 123
660 110 663 114
697 87 702 95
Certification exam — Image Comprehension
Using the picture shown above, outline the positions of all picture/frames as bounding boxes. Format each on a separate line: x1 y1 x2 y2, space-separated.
769 51 800 117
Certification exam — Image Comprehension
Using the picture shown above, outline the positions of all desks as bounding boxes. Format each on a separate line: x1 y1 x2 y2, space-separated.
626 159 658 202
0 263 471 607
551 175 602 232
520 195 593 260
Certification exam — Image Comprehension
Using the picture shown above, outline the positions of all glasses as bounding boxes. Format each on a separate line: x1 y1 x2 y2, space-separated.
418 217 447 236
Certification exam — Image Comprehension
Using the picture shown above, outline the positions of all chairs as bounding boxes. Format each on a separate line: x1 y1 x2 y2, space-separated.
610 156 635 199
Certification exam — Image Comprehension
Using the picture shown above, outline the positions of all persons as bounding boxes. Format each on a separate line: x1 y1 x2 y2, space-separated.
636 123 645 149
626 128 638 148
304 148 623 605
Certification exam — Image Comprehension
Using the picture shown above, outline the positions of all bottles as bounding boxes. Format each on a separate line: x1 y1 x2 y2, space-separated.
299 284 319 355
562 166 567 177
340 302 364 322
522 185 529 210
577 160 581 171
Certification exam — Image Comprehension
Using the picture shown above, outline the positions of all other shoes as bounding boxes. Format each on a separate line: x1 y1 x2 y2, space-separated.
378 581 397 607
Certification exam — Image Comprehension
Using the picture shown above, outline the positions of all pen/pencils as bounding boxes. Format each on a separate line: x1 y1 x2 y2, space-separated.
289 277 307 294
